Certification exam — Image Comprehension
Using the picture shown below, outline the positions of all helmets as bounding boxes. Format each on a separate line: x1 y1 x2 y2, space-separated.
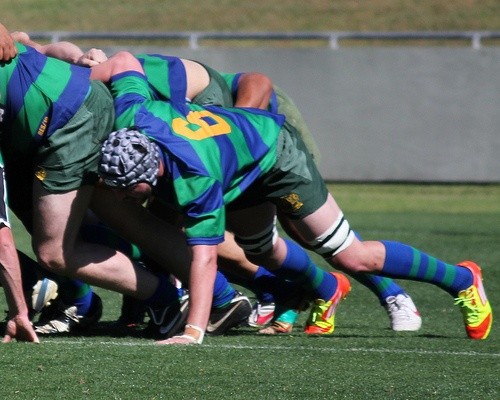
98 128 160 189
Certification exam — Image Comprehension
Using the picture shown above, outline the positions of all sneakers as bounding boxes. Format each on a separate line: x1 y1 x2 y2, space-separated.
246 297 276 328
204 291 252 337
5 274 58 331
383 294 421 332
143 292 192 342
33 292 102 335
259 294 311 334
303 271 351 336
452 259 492 340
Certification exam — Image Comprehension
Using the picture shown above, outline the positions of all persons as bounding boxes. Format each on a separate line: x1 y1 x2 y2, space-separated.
0 23 422 343
89 51 492 342
